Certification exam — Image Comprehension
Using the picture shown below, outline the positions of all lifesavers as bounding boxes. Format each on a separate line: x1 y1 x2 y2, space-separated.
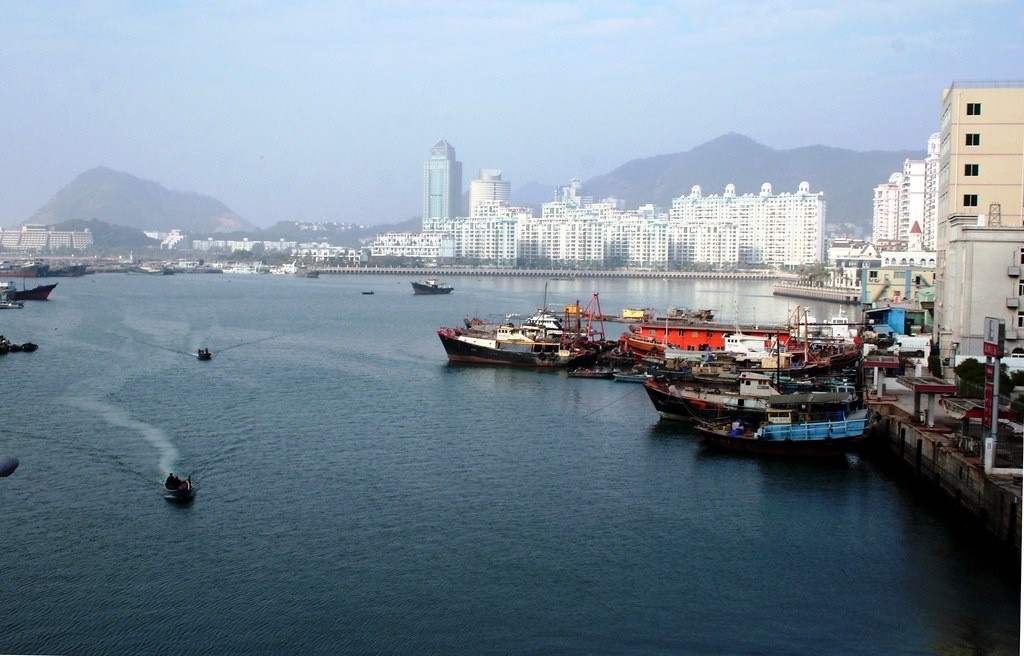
696 388 700 392
539 353 546 361
715 389 720 394
549 354 557 360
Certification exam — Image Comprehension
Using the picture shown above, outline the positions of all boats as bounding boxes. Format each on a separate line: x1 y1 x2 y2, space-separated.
197 353 212 360
0 276 58 309
160 475 198 506
568 368 620 378
613 370 663 384
437 281 617 370
626 305 874 460
410 278 454 295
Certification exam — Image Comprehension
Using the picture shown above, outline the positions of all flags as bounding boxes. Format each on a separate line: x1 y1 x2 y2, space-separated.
439 324 447 330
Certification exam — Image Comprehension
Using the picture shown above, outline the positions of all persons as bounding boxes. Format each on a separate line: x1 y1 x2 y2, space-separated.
165 472 191 490
198 347 209 353
445 315 471 337
